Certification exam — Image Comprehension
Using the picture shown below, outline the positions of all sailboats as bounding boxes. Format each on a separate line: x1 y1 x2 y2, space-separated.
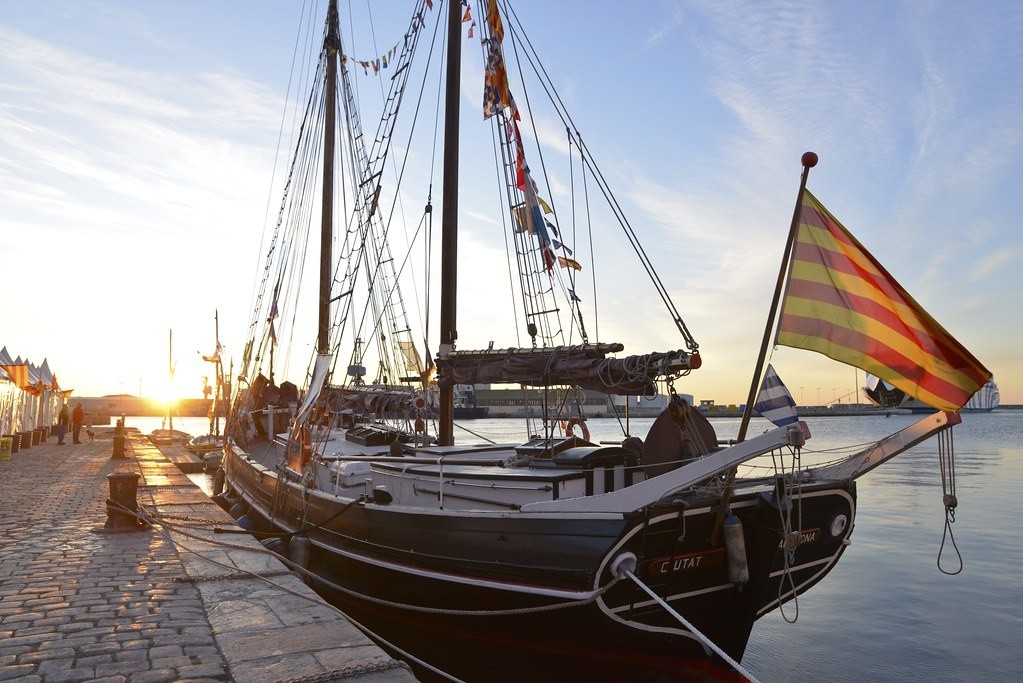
205 0 999 683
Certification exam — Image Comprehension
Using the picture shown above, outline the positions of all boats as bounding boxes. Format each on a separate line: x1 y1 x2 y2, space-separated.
186 433 223 455
150 327 191 445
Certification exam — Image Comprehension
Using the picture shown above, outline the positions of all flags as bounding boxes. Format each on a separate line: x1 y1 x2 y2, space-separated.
461 0 583 277
567 288 582 302
324 0 433 76
0 363 73 399
754 364 799 428
774 187 993 416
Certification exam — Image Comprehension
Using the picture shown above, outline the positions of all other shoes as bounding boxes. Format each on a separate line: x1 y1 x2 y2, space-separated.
73 441 82 444
58 441 66 445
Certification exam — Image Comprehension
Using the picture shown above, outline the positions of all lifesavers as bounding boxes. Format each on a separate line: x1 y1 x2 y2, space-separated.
301 421 313 466
565 418 591 441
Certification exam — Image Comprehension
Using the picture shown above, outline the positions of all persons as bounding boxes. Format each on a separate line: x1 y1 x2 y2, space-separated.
58 403 68 445
72 401 84 444
